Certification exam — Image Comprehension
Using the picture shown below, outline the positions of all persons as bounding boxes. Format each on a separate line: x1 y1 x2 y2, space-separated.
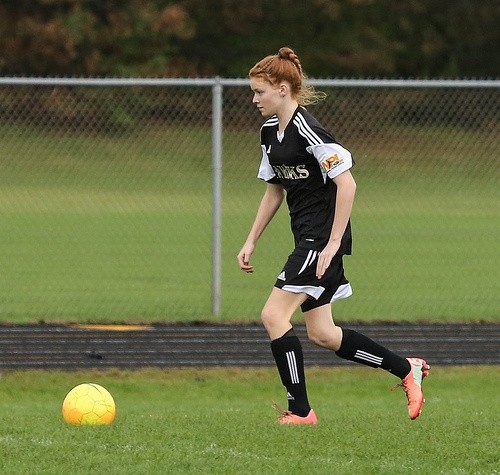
237 48 429 424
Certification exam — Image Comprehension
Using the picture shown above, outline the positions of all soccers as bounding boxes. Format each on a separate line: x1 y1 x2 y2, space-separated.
62 383 116 425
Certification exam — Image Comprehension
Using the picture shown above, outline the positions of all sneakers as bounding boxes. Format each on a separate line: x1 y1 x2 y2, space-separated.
272 400 317 424
391 358 430 420
